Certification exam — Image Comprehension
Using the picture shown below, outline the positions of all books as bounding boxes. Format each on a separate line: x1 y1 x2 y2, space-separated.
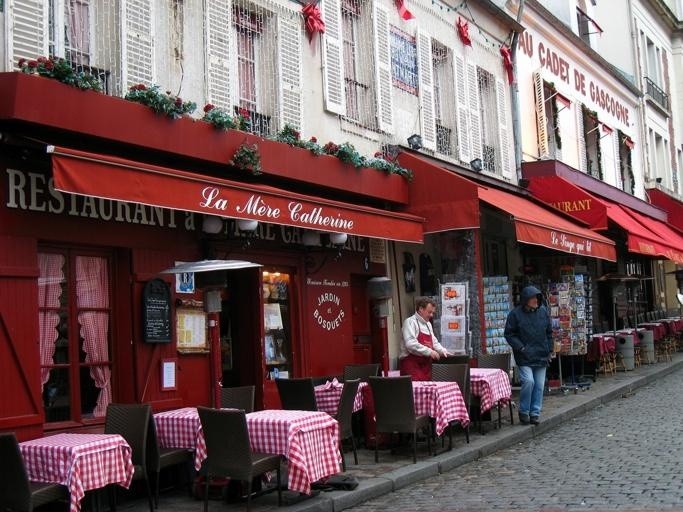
545 273 593 356
482 276 515 355
263 302 287 365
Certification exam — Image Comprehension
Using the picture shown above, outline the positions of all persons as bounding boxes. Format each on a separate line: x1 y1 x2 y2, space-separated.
398 295 455 442
504 285 554 426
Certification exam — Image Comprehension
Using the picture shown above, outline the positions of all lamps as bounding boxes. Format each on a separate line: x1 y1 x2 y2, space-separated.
329 233 347 263
407 134 422 149
470 158 482 172
238 220 258 252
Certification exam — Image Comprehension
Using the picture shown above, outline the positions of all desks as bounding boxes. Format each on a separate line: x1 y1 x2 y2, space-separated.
586 309 683 377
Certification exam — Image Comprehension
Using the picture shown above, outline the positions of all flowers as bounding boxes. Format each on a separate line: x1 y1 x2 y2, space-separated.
17 54 104 92
124 83 196 121
274 123 414 183
199 103 253 132
228 139 263 176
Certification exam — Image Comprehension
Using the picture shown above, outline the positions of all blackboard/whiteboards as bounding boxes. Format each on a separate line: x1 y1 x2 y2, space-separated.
144 296 171 343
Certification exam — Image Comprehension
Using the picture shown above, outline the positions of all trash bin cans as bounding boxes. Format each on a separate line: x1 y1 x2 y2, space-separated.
616 335 634 370
638 330 654 364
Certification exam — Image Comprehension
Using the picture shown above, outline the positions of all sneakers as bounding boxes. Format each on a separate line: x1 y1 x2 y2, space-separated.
519 412 529 423
530 416 540 424
